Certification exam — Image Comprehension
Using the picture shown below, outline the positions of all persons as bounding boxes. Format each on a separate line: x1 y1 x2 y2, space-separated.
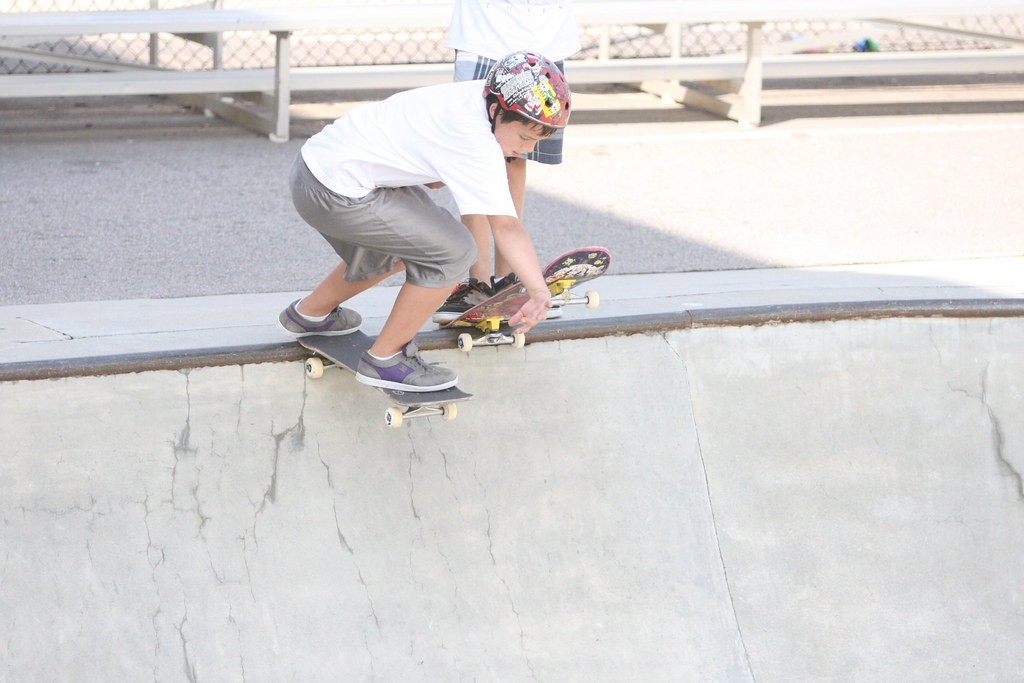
433 2 580 323
275 49 571 392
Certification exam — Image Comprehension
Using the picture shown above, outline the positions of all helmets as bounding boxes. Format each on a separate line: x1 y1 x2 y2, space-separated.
484 52 572 129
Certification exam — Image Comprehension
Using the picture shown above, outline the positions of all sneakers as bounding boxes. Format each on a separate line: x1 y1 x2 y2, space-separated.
355 338 458 392
432 278 496 324
278 298 363 337
491 272 561 318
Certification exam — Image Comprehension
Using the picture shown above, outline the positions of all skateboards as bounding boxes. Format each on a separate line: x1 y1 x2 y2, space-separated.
442 246 613 352
297 329 473 427
